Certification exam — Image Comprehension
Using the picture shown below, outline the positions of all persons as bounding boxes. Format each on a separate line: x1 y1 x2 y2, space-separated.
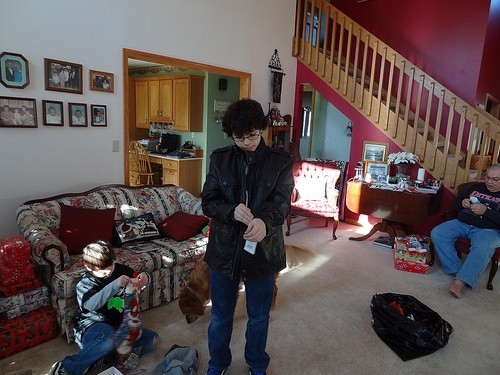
431 164 500 298
200 98 295 375
1 62 110 124
48 240 158 375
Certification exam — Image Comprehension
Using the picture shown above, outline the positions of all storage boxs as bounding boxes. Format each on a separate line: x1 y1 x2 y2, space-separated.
0 236 58 360
394 259 428 274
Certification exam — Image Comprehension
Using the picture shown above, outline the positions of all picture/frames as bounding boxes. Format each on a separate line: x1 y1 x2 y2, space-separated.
0 51 30 89
44 57 84 94
90 70 114 93
0 96 107 128
362 141 390 177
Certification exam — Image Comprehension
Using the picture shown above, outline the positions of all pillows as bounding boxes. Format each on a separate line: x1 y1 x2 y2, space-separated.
157 211 209 242
58 202 115 254
111 213 161 247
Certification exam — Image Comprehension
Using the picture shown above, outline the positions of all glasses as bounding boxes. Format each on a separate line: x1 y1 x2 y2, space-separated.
484 175 500 184
232 128 262 142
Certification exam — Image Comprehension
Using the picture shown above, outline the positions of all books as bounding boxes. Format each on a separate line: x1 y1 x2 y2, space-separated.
122 241 162 252
373 236 394 248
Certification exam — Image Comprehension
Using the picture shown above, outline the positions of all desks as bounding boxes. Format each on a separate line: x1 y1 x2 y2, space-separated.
345 177 442 246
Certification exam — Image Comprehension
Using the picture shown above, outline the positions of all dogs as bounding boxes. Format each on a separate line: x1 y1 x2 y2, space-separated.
177 242 316 325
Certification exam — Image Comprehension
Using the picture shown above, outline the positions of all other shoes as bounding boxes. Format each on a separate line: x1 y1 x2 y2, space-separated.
248 367 266 375
207 365 226 375
49 360 68 375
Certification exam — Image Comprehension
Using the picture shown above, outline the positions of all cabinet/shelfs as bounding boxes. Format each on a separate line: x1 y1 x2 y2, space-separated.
261 115 292 152
128 75 204 140
129 152 202 198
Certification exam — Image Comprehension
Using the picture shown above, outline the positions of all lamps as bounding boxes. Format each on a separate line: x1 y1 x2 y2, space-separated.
344 121 352 137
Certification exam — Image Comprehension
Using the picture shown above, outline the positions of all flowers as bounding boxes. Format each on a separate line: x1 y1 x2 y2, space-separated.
387 152 420 168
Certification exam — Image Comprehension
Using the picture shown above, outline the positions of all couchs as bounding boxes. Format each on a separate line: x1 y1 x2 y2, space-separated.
15 183 210 345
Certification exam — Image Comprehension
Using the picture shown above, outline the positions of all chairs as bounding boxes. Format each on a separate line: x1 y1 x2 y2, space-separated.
285 161 341 241
129 141 155 185
454 183 500 290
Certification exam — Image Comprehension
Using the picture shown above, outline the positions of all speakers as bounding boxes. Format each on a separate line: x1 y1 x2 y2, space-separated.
219 79 227 90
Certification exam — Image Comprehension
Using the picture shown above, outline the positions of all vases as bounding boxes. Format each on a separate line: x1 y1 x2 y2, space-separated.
396 164 408 175
